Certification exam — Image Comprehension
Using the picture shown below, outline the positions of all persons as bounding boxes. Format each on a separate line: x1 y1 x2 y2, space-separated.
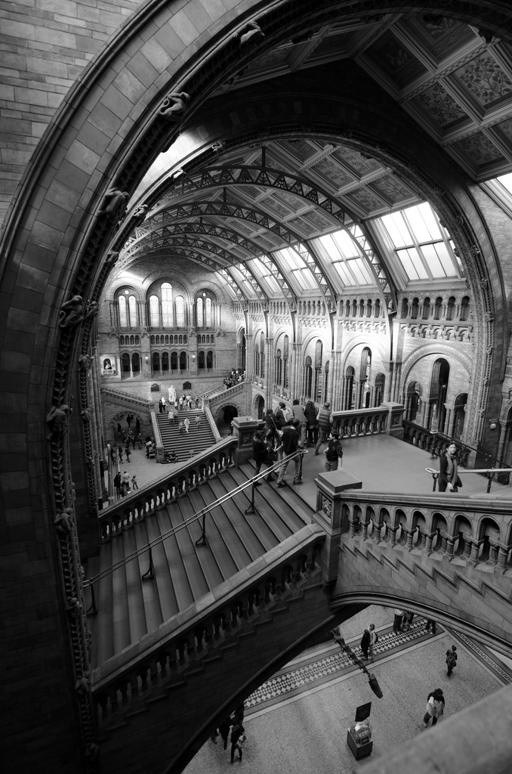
105 368 245 499
361 609 457 728
436 442 463 493
252 399 343 488
211 700 247 762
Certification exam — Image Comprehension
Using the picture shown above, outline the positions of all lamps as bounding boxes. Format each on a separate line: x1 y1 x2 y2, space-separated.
191 354 197 363
144 354 150 364
487 417 498 431
